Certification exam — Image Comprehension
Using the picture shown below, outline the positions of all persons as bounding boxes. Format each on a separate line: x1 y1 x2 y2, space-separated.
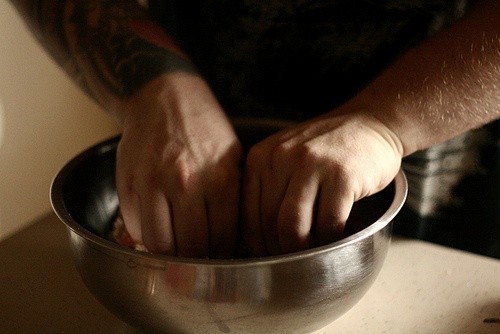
10 0 500 258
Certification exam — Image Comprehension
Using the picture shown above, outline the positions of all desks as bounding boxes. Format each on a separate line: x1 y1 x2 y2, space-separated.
0 211 500 334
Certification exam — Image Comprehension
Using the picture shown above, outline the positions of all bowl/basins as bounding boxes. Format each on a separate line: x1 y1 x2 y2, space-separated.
49 116 409 334
